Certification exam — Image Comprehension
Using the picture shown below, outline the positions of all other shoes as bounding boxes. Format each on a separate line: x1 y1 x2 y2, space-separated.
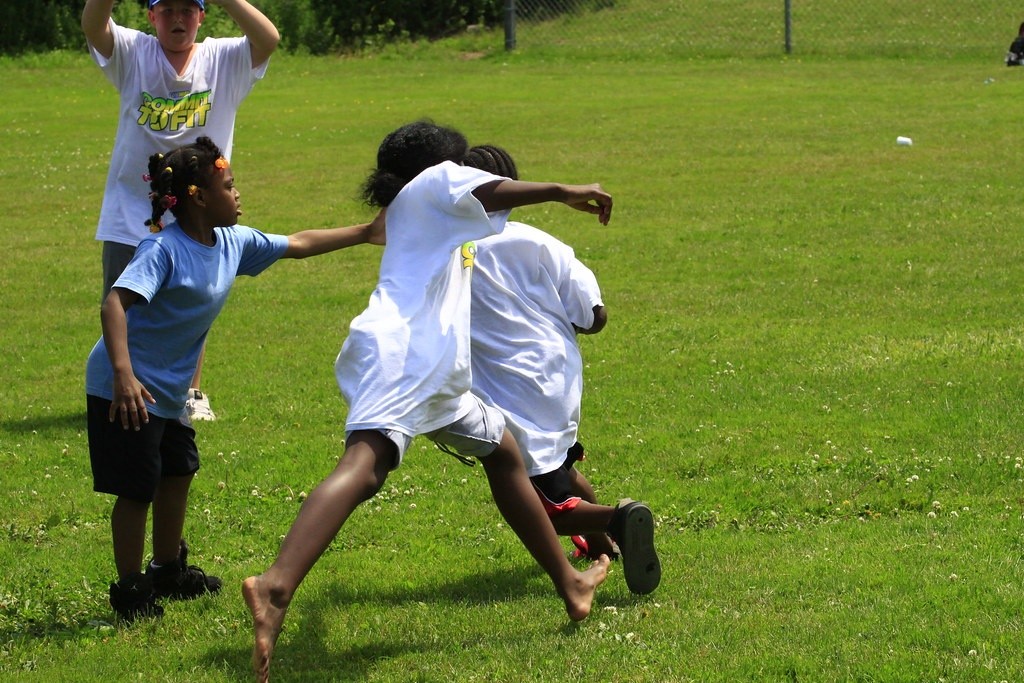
607 497 661 595
570 535 618 561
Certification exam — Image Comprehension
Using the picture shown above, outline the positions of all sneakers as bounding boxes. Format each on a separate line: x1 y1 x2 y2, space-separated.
179 387 215 423
108 582 164 621
145 540 221 599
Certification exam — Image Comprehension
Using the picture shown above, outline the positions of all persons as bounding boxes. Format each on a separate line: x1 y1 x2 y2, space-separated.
82 1 280 422
1003 21 1024 66
242 119 614 683
463 145 661 594
84 135 387 626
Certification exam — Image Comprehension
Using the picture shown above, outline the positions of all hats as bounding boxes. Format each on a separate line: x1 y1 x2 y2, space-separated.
149 0 204 11
